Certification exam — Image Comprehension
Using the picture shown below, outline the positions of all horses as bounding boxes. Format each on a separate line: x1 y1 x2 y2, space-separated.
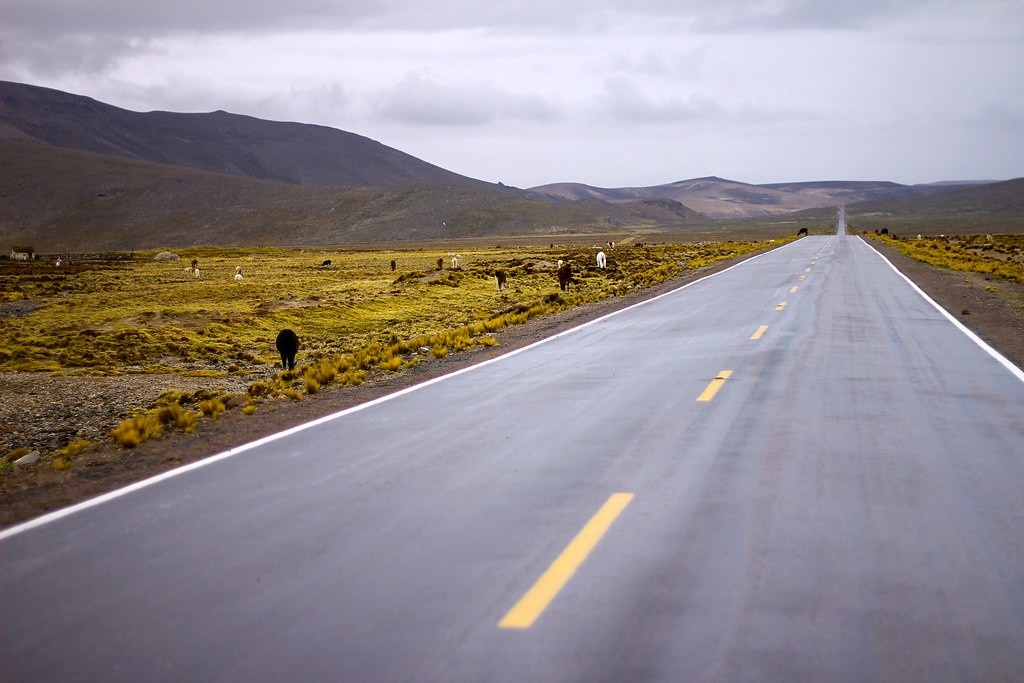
234 266 244 281
596 251 606 268
797 228 808 236
321 260 331 266
190 259 197 268
437 258 443 269
557 259 571 292
494 270 508 292
451 255 462 268
276 329 299 371
391 261 396 271
880 228 888 236
